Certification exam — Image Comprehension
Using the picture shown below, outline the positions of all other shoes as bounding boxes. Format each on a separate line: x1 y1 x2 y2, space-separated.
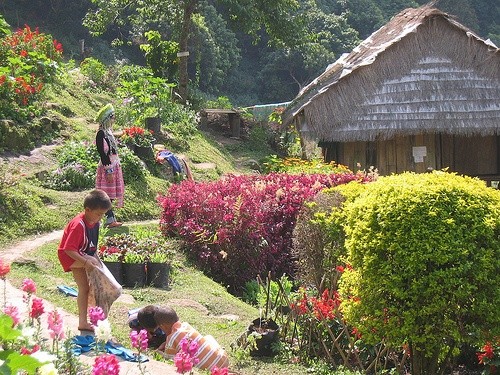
106 222 121 227
115 223 122 226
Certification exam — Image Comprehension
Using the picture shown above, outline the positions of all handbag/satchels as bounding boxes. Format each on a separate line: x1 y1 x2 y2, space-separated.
85 252 123 322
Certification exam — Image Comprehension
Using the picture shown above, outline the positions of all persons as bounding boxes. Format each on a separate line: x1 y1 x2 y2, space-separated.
94 103 125 228
58 189 123 330
127 303 229 373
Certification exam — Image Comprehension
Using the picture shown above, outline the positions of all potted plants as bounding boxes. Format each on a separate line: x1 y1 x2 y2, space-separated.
100 249 175 289
246 276 293 356
